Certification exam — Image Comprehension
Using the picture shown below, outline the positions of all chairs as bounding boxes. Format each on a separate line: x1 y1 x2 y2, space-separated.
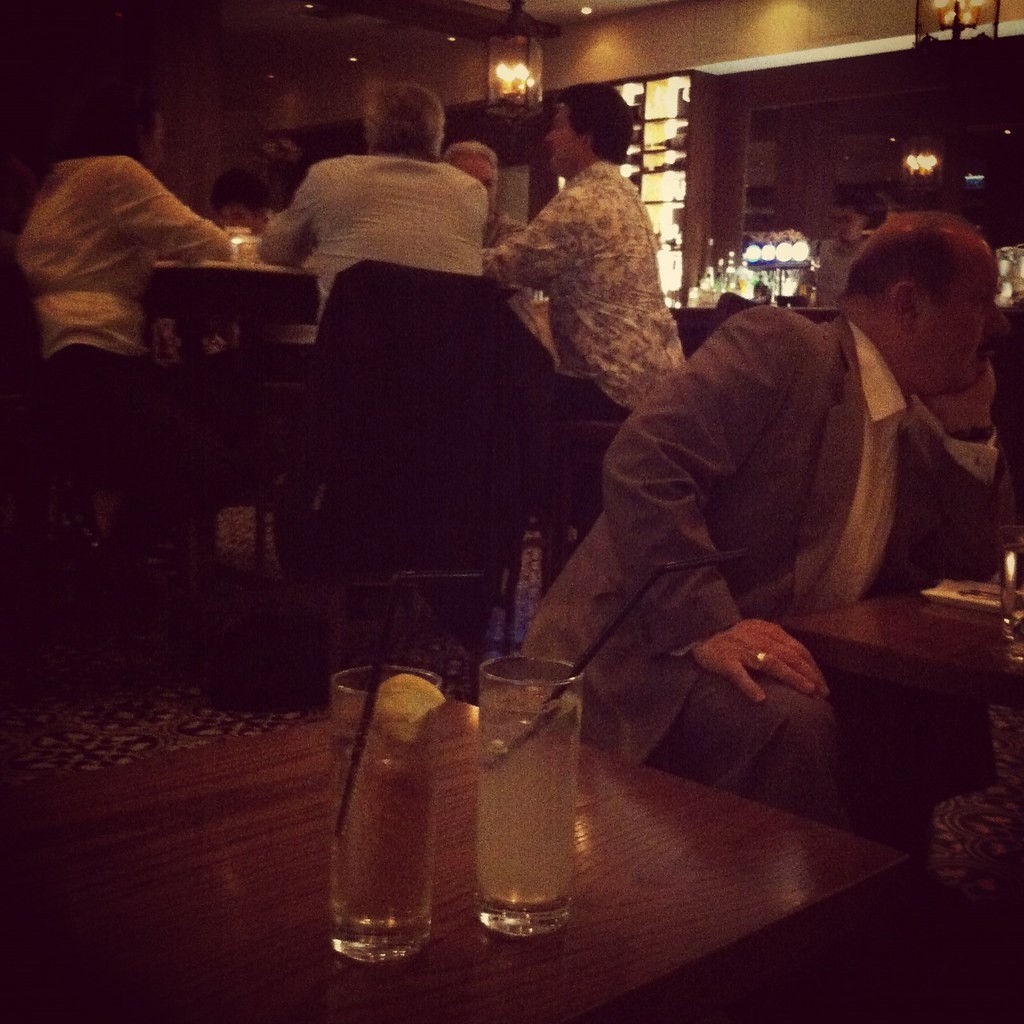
53 259 651 701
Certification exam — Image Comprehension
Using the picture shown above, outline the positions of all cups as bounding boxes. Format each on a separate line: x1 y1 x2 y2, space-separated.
473 655 585 936
322 665 443 964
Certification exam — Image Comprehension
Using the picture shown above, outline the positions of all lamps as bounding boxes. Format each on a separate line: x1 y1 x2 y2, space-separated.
914 0 1001 72
486 0 545 108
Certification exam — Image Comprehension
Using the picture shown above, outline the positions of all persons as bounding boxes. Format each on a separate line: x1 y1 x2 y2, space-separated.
258 80 488 326
794 186 883 308
482 85 687 583
444 141 530 248
16 83 236 587
518 211 1013 853
209 168 271 234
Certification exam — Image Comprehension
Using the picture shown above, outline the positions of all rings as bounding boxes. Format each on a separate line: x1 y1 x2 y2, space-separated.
755 652 768 670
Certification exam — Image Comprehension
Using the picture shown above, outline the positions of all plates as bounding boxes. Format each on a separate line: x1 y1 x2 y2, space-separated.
920 578 1024 613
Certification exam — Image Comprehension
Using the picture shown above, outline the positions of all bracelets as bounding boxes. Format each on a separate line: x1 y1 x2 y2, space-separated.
948 425 992 443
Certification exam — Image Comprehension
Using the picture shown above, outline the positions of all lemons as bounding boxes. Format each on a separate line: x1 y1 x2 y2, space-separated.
373 673 446 745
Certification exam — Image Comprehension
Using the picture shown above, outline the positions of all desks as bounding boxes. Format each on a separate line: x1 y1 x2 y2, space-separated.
780 561 1024 927
0 705 907 1024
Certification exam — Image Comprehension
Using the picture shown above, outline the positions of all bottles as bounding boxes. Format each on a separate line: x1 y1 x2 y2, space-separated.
699 237 766 299
231 228 252 264
